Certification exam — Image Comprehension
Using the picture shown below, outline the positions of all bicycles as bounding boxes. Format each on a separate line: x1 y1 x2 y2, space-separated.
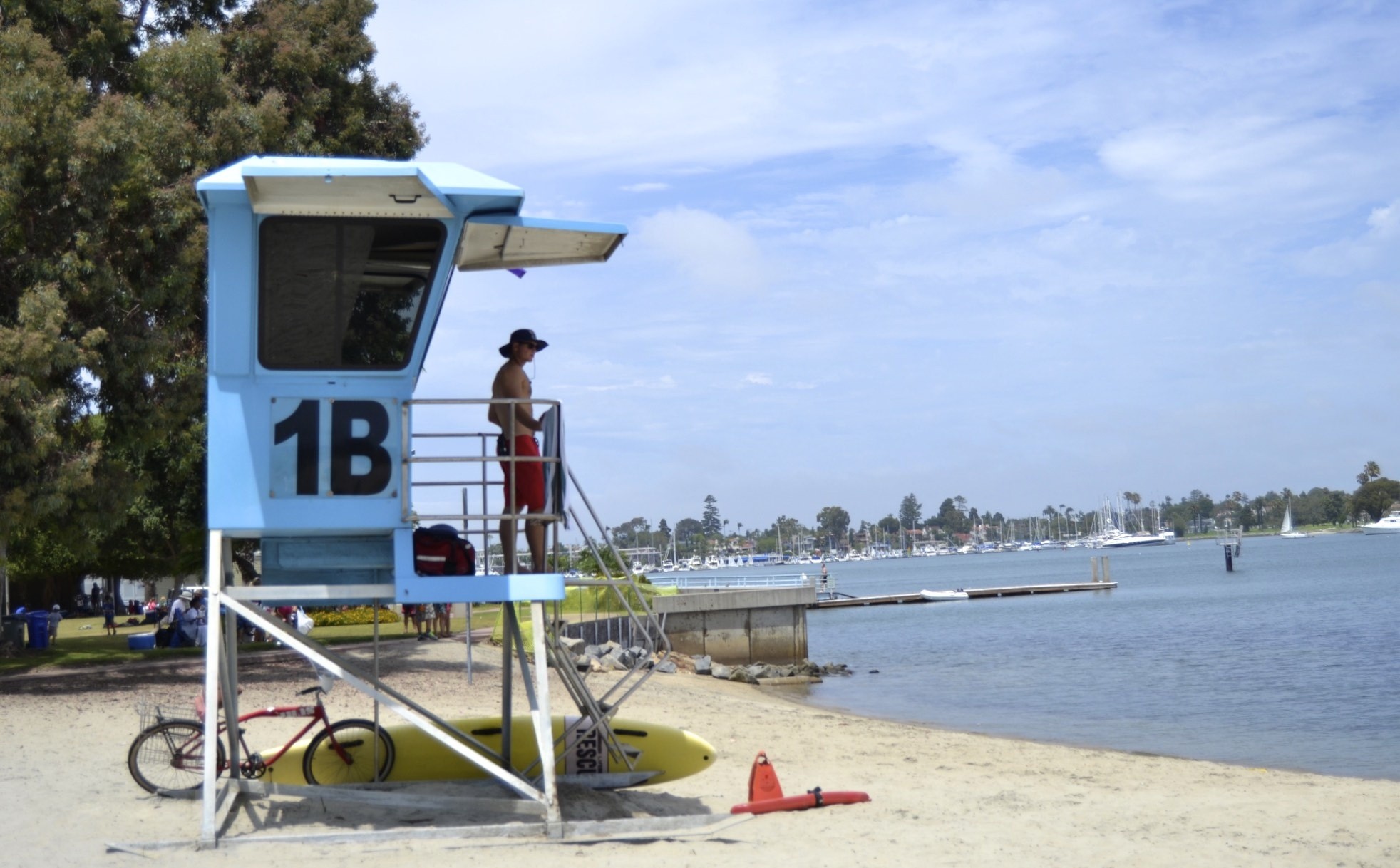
127 680 397 799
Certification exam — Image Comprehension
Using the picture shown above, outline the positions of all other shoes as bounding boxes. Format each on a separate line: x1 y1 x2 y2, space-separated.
417 634 430 640
425 632 438 639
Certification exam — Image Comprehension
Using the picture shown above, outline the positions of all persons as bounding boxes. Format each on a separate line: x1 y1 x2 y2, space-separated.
487 329 549 572
401 602 453 640
820 564 827 592
89 582 300 647
47 604 63 646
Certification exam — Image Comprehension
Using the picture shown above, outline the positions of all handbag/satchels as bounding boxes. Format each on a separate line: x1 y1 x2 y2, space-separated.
412 524 475 575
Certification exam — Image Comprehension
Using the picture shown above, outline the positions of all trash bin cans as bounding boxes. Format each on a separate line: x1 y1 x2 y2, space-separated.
26 610 51 649
3 614 26 646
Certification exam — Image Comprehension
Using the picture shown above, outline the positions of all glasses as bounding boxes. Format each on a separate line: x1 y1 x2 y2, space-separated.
518 342 541 351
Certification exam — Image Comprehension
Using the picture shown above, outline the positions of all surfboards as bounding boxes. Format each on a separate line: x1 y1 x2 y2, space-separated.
256 713 720 789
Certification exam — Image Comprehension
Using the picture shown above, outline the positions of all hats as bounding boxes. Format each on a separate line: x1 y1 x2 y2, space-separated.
499 330 549 358
179 592 193 601
53 604 60 610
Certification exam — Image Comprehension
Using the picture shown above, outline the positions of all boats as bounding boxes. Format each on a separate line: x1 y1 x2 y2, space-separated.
1356 510 1400 535
920 588 970 602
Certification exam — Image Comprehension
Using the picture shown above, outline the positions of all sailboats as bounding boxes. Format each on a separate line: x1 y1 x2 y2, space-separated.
566 526 754 578
763 494 1177 565
1278 496 1309 540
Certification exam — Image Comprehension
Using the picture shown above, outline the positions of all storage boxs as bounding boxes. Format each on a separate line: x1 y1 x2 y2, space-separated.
128 632 156 650
